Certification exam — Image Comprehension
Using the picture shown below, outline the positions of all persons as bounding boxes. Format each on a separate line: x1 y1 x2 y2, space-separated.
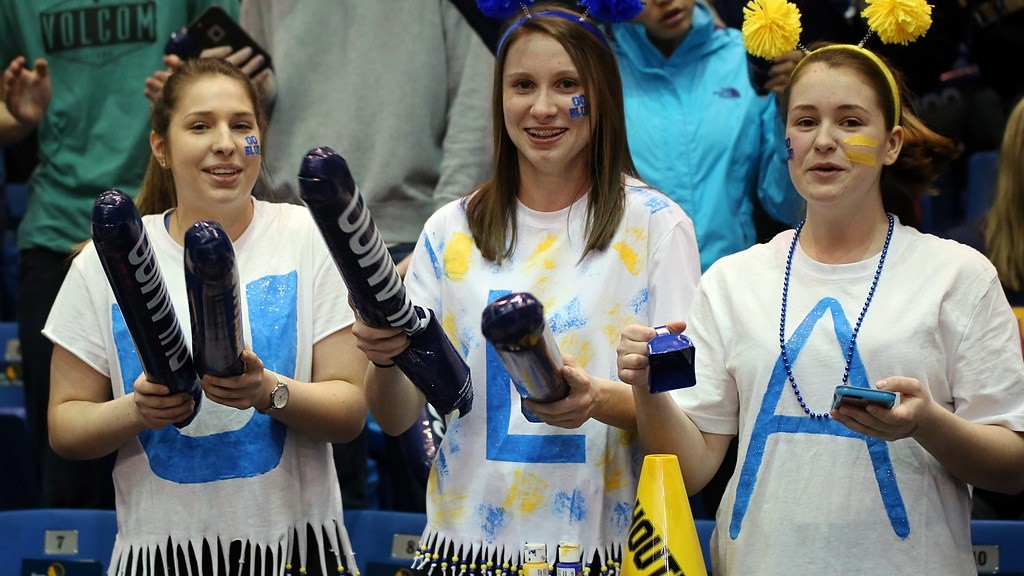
42 55 384 576
0 0 1022 404
617 46 1024 576
347 4 700 575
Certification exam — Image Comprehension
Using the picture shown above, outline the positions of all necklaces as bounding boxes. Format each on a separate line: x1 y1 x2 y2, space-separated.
776 207 894 421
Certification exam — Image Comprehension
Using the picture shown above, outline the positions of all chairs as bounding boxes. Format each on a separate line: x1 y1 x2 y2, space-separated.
0 321 1024 575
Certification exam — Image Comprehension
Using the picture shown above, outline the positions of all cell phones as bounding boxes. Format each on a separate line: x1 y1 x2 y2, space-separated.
830 384 895 417
188 6 271 76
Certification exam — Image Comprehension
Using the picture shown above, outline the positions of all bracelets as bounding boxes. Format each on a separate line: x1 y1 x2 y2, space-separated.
370 359 396 369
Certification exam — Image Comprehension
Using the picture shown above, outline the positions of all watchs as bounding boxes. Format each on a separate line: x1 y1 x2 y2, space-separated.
256 373 290 415
